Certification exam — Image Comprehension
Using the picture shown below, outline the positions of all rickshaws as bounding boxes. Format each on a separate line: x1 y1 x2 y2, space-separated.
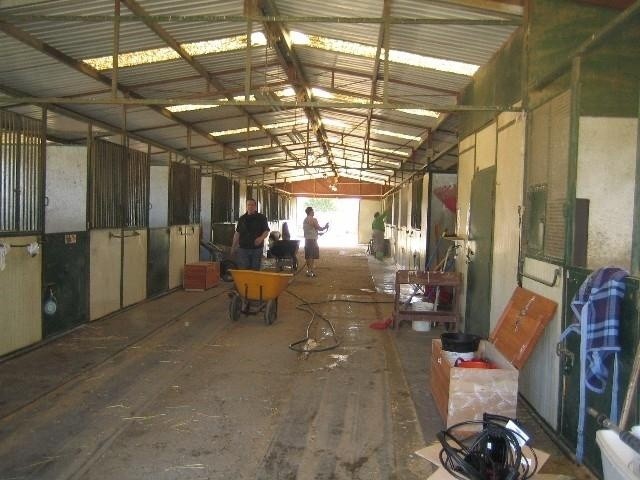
227 269 294 323
271 240 299 270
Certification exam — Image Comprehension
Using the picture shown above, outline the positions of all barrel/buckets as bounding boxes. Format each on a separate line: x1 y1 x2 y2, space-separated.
375 251 383 259
412 302 434 331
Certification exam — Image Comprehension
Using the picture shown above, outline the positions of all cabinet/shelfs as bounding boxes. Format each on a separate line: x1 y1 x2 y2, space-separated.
393 269 463 335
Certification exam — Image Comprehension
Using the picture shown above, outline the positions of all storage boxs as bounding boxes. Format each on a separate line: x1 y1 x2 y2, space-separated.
427 285 558 432
184 260 220 291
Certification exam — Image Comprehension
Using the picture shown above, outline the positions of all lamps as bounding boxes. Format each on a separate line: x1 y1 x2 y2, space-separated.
288 106 305 145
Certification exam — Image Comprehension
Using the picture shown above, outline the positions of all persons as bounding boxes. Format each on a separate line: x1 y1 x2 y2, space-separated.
302 207 330 278
229 197 270 271
372 204 393 261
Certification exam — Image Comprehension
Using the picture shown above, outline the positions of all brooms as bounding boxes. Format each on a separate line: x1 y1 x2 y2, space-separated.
369 257 446 329
392 228 448 312
